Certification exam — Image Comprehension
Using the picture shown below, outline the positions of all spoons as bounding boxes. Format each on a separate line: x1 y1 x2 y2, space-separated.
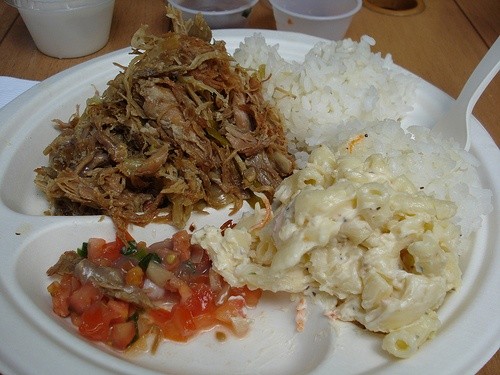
431 36 500 164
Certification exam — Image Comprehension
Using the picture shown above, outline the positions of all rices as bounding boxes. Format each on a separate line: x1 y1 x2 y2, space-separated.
234 31 493 239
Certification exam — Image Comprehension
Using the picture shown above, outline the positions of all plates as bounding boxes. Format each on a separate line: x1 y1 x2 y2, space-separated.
0 28 500 375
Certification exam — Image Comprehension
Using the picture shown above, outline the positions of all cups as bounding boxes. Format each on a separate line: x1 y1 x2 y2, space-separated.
14 0 116 59
168 0 259 30
270 0 362 40
